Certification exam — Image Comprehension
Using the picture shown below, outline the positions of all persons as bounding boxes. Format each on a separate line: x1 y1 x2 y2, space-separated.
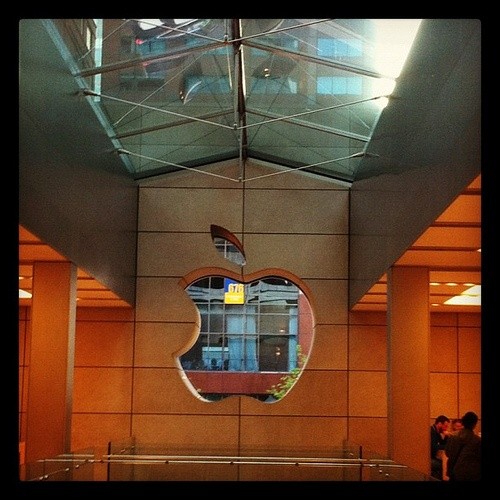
440 410 482 482
429 415 451 480
446 417 463 434
